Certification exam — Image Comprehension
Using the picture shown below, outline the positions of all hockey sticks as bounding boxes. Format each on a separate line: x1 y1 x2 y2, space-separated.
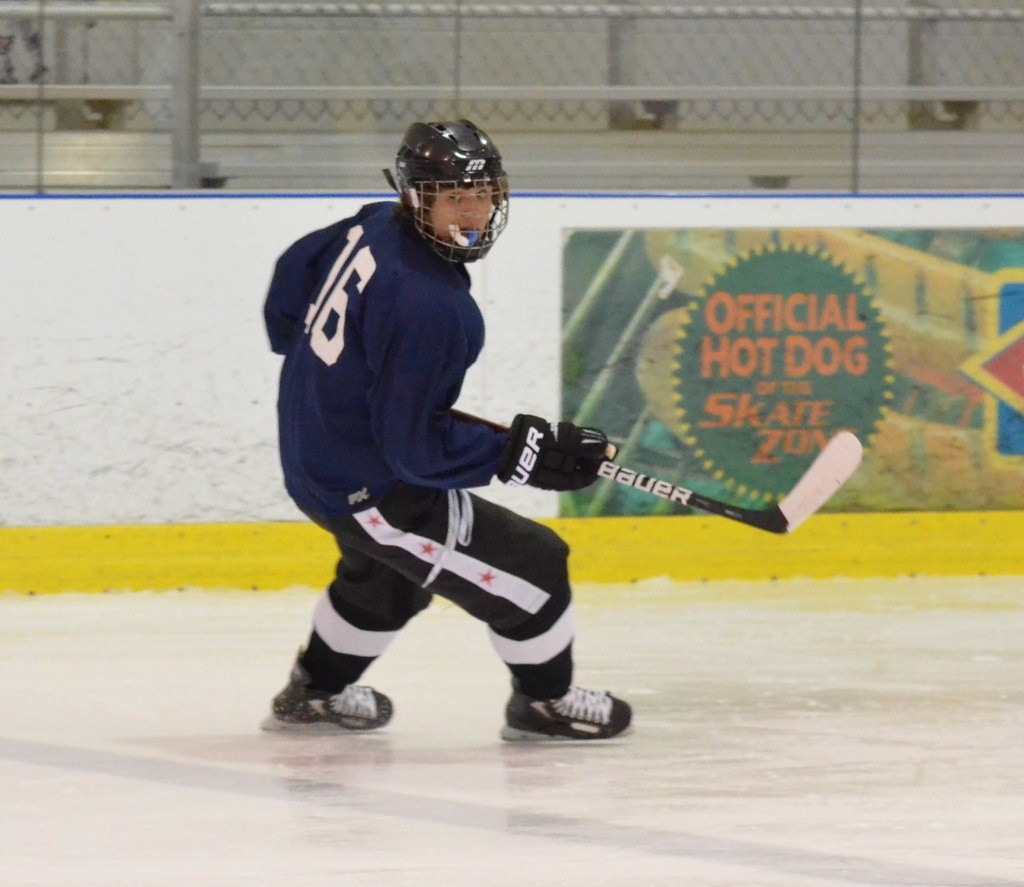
593 429 865 534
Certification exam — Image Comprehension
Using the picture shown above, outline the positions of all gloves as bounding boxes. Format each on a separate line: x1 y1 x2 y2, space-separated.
498 414 618 491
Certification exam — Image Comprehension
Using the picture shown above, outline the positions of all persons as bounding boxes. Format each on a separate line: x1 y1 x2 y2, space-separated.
264 118 632 741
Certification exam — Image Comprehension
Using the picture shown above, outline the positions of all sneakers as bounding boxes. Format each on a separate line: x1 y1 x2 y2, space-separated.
499 681 633 742
271 647 394 729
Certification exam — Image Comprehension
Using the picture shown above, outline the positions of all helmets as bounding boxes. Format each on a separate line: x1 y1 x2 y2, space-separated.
395 118 510 265
